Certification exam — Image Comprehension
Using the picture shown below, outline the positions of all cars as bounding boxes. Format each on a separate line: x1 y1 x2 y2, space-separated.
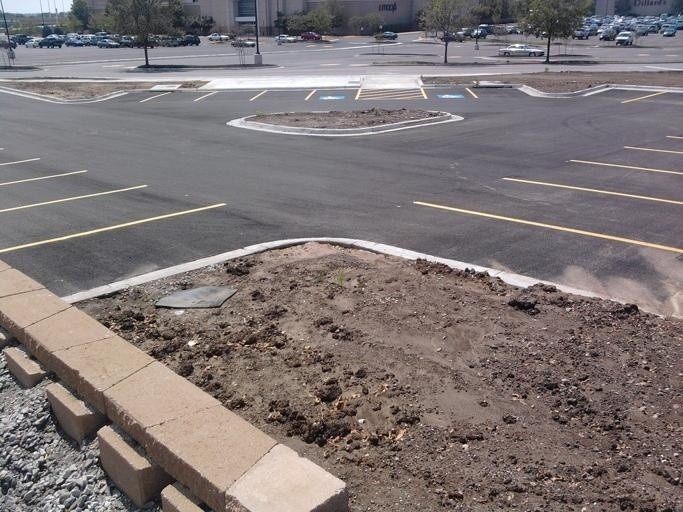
499 44 544 57
12 31 199 49
572 13 683 46
456 23 516 38
301 32 321 40
207 32 230 41
374 31 397 40
275 34 297 43
232 38 254 47
